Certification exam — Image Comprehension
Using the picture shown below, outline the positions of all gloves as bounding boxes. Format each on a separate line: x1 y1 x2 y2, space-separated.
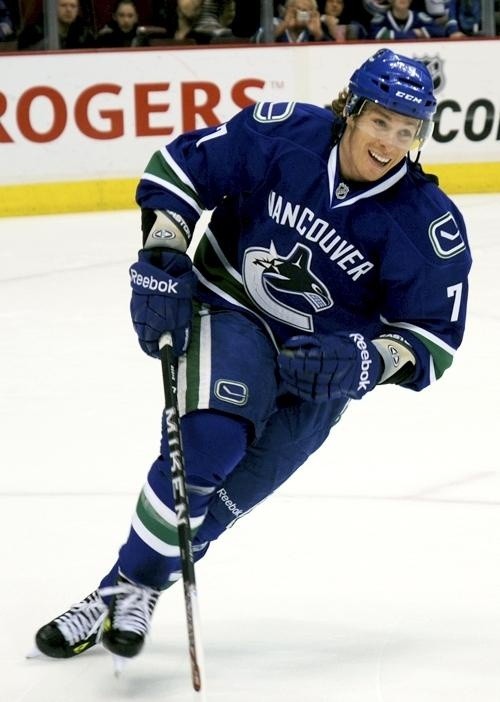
129 246 195 362
278 333 382 404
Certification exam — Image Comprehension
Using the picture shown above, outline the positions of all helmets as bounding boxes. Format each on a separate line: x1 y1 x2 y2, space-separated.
345 47 438 137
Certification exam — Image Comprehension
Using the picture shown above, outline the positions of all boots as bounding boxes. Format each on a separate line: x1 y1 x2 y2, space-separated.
101 570 163 658
36 587 110 659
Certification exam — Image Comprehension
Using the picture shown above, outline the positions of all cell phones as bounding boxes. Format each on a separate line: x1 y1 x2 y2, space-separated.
321 14 339 26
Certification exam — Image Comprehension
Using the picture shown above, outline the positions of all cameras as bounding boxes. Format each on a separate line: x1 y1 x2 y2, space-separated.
296 11 310 20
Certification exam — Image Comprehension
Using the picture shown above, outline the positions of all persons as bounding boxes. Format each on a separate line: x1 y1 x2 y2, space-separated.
35 49 472 663
0 0 500 53
